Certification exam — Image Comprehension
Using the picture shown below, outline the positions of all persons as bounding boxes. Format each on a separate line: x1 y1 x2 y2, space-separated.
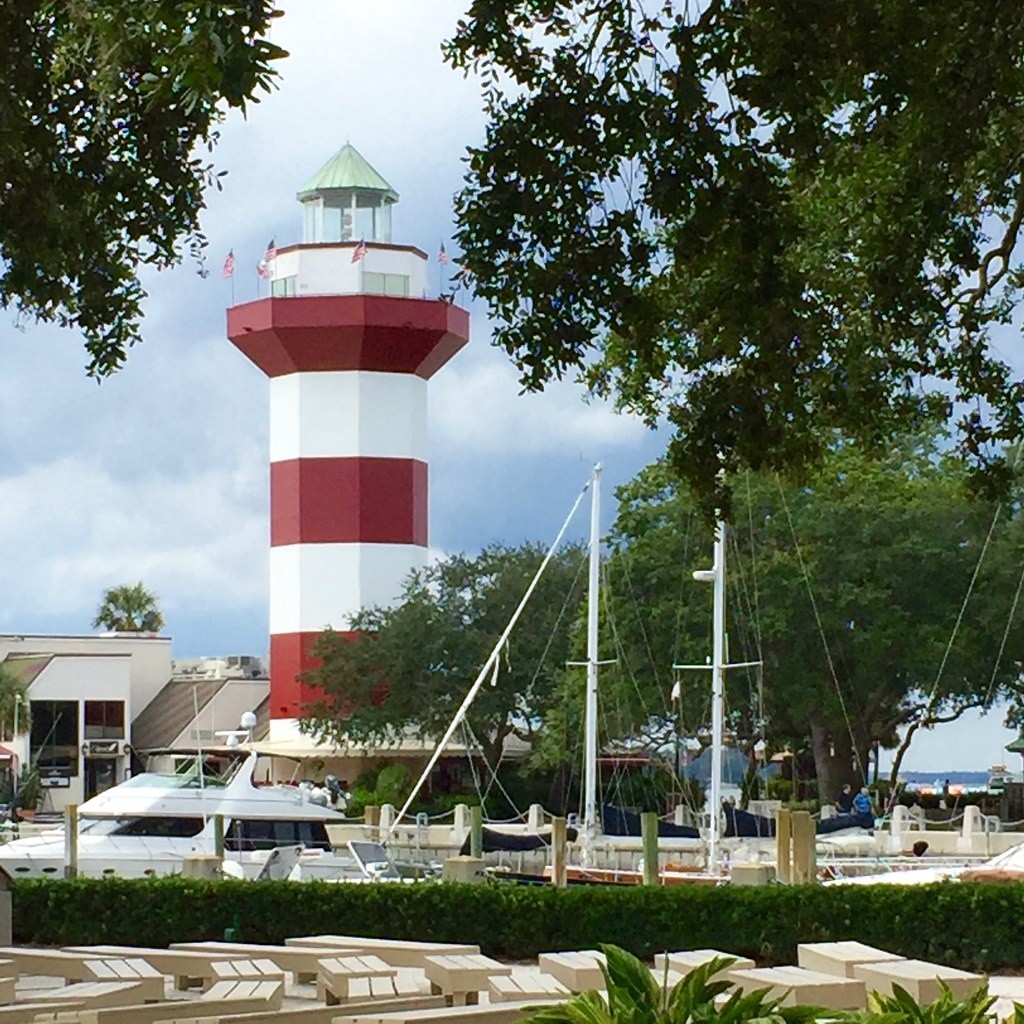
943 779 949 794
853 788 872 814
836 784 852 816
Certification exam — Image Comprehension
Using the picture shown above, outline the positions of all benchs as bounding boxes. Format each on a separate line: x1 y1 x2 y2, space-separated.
0 935 993 1024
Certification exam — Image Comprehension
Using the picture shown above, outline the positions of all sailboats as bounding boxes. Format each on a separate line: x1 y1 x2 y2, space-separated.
355 457 1024 885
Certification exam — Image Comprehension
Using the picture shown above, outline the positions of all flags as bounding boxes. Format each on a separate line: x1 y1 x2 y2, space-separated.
264 240 275 262
439 245 447 265
258 266 268 279
224 252 234 278
352 240 367 263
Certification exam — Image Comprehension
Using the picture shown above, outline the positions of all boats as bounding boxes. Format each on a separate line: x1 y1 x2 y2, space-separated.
0 683 400 883
